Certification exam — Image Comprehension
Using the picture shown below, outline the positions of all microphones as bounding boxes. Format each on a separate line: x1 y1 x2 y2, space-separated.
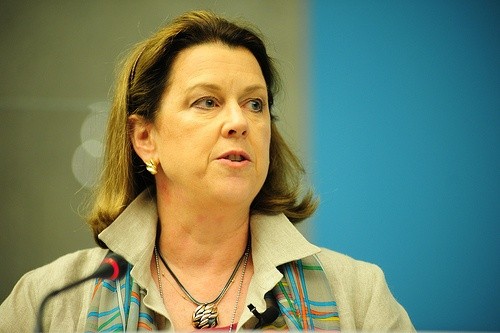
248 303 280 329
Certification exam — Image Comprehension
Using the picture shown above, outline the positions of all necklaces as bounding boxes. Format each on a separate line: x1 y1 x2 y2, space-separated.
153 237 254 329
152 247 250 332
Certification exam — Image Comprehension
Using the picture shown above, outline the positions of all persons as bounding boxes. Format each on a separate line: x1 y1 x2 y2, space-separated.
1 9 419 333
